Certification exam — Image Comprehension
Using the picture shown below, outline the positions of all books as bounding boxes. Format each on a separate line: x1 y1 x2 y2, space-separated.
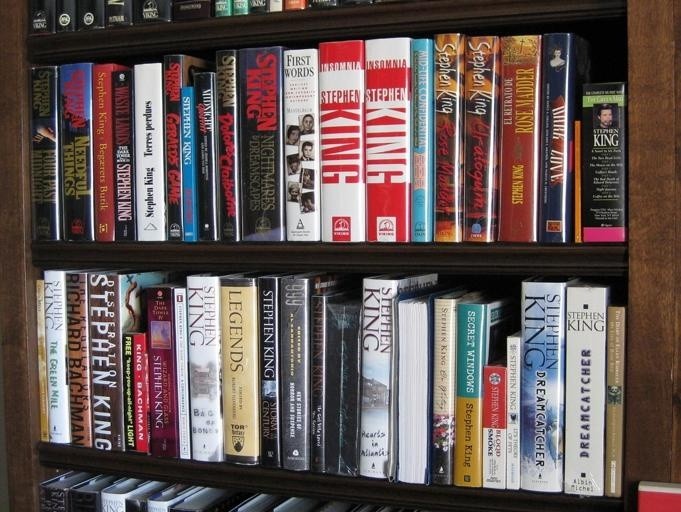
39 472 427 512
37 266 626 497
24 33 629 244
26 1 382 37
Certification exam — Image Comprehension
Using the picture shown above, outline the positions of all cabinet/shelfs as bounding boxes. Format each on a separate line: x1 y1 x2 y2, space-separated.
0 0 681 512
